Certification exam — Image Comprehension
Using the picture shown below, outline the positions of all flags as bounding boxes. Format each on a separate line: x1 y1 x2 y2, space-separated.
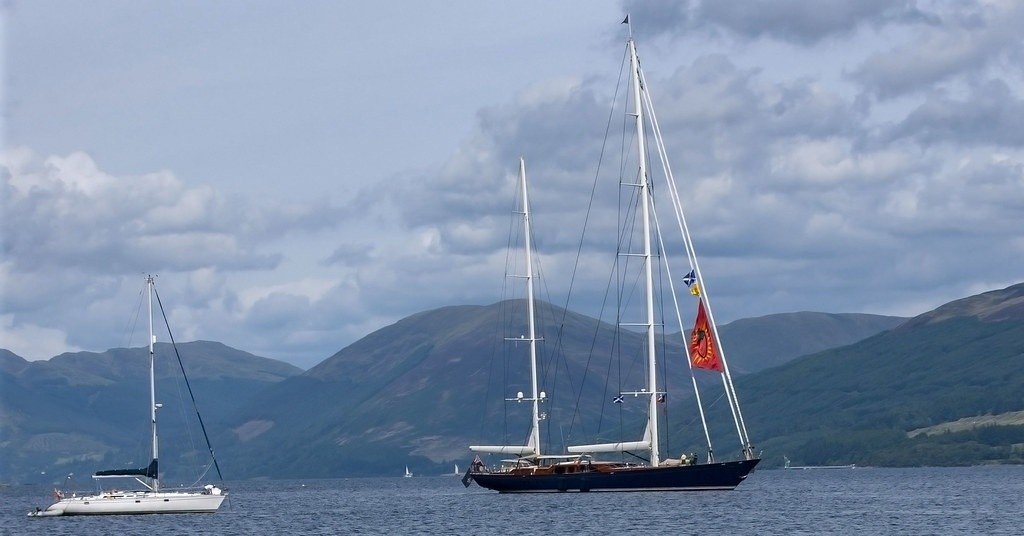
461 455 484 488
657 395 665 403
682 269 699 296
613 395 624 403
689 299 724 372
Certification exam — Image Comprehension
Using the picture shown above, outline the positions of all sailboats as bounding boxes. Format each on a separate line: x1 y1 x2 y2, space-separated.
453 464 461 477
47 272 233 516
461 14 763 494
404 465 413 478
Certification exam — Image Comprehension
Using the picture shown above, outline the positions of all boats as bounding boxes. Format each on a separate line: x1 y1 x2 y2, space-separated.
27 506 65 517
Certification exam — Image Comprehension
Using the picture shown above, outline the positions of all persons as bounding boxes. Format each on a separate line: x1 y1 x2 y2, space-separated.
681 453 698 465
